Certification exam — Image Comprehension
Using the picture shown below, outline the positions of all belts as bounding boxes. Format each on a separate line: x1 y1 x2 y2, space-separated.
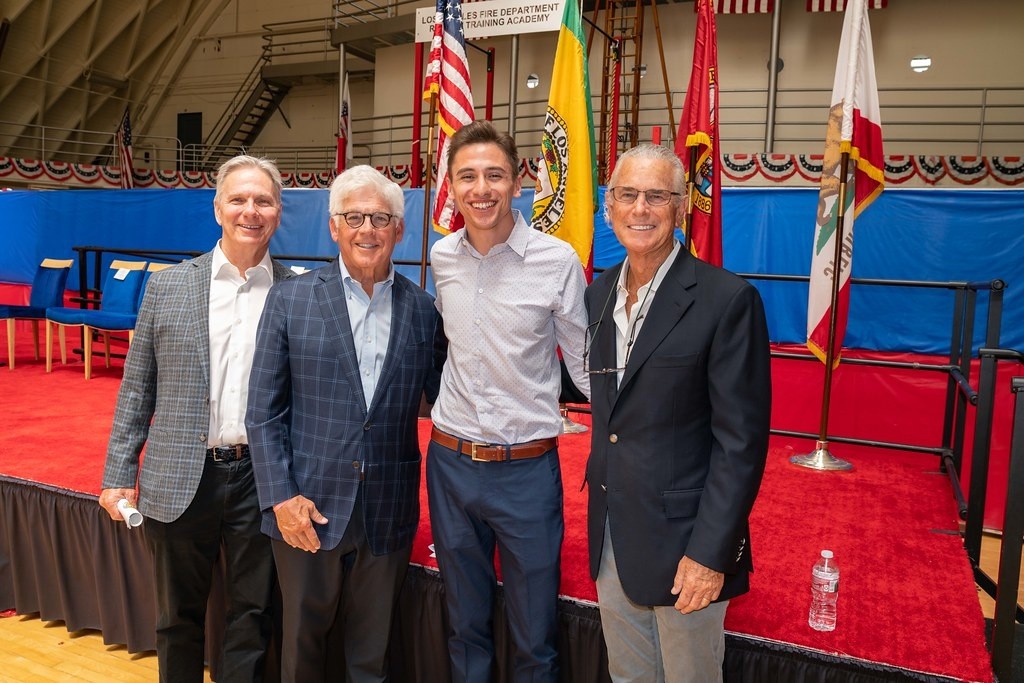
430 424 559 462
206 444 250 463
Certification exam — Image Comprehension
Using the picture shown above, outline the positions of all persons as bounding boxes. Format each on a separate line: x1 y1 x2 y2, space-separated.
98 156 297 682
559 144 773 683
429 122 591 683
244 164 448 682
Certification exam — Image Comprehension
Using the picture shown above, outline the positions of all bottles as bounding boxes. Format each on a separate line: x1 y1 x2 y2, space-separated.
809 550 839 631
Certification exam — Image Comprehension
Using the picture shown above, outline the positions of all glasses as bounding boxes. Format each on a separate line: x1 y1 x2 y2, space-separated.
334 211 399 229
581 313 644 374
610 185 681 206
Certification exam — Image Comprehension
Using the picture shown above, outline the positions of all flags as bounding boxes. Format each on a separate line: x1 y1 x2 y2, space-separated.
334 80 353 170
422 1 475 238
806 0 886 372
675 1 722 269
531 0 599 360
116 105 134 190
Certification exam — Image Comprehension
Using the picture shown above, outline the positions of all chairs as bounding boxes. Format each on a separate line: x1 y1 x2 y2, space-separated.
0 258 74 371
82 262 181 380
45 260 148 380
